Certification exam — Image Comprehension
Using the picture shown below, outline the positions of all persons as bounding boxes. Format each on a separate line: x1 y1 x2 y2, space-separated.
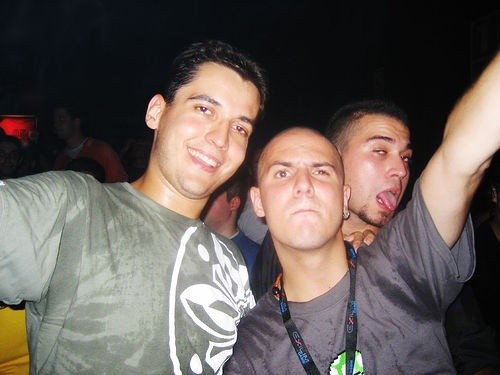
0 105 152 188
0 40 269 375
223 52 500 375
248 99 413 303
199 143 282 300
442 150 500 375
